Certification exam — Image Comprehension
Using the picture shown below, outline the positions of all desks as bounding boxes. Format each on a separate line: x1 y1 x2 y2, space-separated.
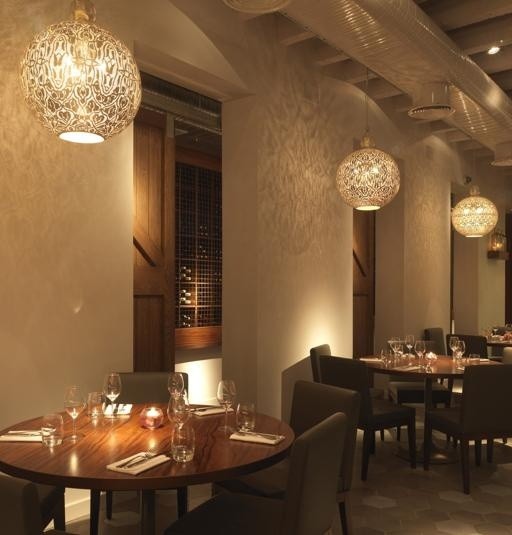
358 355 502 465
0 404 296 535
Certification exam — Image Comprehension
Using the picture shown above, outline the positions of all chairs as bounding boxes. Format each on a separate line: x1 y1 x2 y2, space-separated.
319 354 418 482
468 346 511 464
162 412 346 535
424 327 444 354
424 364 512 495
37 483 66 531
382 341 444 443
484 337 512 357
310 345 384 455
89 370 189 535
212 378 360 535
0 470 79 535
493 327 506 337
445 334 487 409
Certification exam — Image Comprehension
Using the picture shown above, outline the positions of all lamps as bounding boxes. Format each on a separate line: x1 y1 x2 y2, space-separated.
335 67 400 211
487 45 500 56
17 0 143 144
488 227 509 251
451 186 498 237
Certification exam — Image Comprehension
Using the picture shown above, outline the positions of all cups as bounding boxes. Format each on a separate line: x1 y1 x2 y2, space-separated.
469 353 480 365
171 427 196 464
236 403 256 432
63 388 87 441
217 379 237 432
168 373 184 399
166 395 191 449
390 337 401 361
450 337 459 361
405 335 414 354
455 340 465 370
41 413 63 447
86 392 103 417
414 340 426 367
103 376 122 423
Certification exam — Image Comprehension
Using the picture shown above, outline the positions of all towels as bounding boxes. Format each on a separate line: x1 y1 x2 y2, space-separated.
359 358 380 361
392 365 420 371
104 404 132 418
106 452 170 476
463 358 489 362
229 430 285 445
0 430 43 442
187 405 234 416
